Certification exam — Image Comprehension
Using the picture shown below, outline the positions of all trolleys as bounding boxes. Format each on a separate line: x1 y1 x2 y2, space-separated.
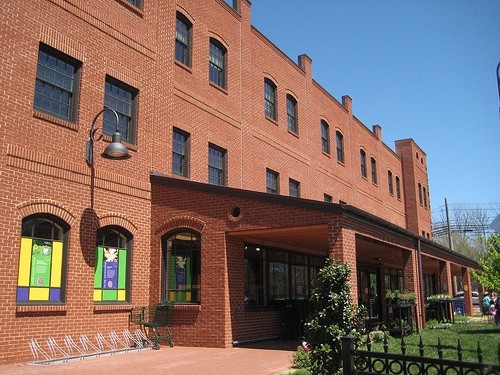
128 299 176 350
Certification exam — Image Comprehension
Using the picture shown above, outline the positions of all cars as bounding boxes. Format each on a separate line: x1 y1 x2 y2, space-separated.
457 290 479 305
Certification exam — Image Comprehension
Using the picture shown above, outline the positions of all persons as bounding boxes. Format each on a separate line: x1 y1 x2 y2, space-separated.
494 295 500 326
490 291 497 315
481 292 491 315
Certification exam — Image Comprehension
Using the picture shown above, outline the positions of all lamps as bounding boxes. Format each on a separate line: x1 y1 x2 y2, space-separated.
86 108 131 165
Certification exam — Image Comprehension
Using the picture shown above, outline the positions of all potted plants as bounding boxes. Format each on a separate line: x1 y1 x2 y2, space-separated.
426 293 455 306
385 289 415 304
